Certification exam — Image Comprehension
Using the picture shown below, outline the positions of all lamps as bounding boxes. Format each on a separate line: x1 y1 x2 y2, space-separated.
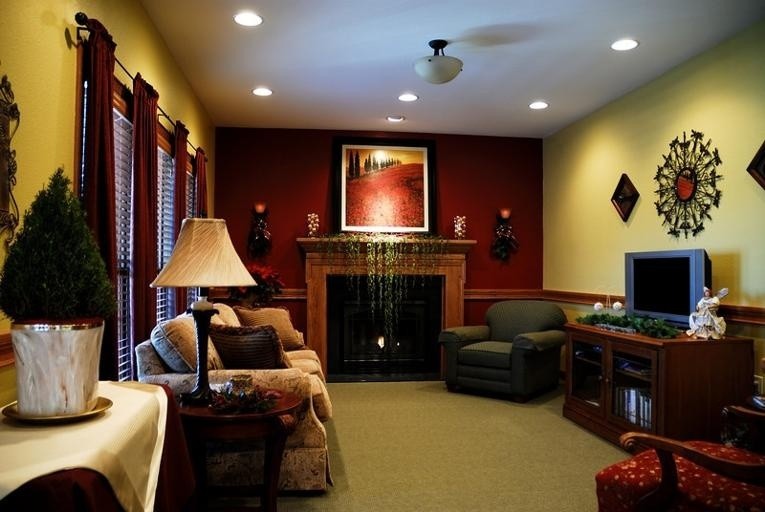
145 214 261 406
413 37 466 84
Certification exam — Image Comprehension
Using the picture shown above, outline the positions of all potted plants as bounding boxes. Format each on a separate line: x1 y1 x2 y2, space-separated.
0 164 118 426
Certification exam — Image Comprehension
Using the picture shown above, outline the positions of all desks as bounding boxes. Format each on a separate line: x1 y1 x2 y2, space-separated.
174 388 305 512
0 381 193 511
721 392 765 453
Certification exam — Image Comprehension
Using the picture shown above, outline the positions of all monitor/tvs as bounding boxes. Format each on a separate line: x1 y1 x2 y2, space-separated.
624 249 712 329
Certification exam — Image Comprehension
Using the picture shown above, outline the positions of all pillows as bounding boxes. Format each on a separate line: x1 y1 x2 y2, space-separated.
210 321 294 373
234 304 305 352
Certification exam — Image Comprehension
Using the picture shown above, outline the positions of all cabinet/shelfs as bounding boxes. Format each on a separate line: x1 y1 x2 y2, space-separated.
562 316 756 458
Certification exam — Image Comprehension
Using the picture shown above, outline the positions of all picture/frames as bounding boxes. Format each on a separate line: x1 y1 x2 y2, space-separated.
610 170 642 224
330 133 440 236
745 134 765 191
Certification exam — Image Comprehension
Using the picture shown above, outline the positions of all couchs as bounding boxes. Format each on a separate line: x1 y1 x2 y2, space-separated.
132 302 337 496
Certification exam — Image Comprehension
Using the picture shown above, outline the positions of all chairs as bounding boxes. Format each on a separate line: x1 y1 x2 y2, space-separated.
589 402 765 512
437 297 569 400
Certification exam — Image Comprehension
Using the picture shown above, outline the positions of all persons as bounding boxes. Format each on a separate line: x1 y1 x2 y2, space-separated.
687 287 729 339
